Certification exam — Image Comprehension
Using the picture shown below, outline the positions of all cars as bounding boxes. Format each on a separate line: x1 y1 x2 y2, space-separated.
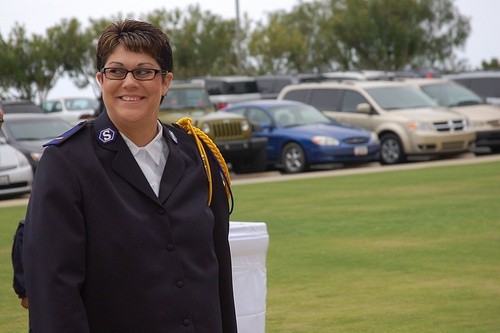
41 92 103 124
0 112 75 172
0 125 35 201
218 98 382 176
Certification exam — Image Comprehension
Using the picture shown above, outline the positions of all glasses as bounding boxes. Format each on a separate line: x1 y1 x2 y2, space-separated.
100 66 168 82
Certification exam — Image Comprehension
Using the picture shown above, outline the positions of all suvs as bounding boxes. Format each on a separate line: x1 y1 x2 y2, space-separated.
156 83 268 165
169 68 441 114
0 99 46 114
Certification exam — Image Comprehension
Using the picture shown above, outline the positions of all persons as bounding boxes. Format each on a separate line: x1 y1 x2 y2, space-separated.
11 220 29 309
23 19 238 333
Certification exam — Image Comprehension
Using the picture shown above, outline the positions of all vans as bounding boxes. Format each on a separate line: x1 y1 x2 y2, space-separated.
396 76 500 154
277 80 472 167
442 71 500 110
